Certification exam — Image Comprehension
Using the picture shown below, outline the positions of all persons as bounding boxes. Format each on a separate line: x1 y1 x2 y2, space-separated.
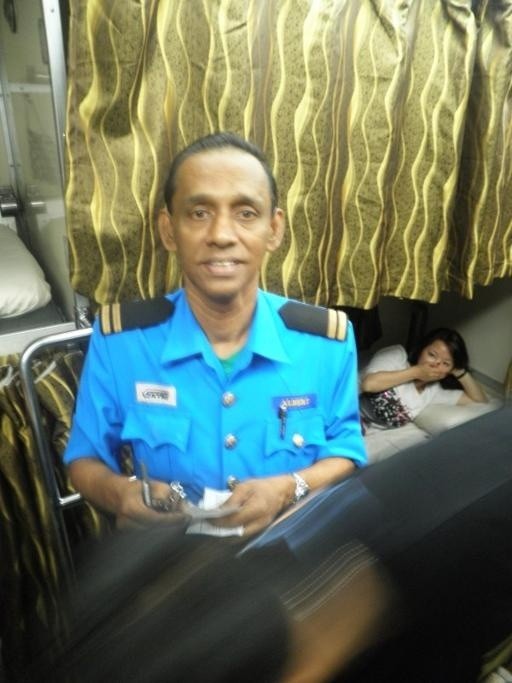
356 325 491 439
64 129 373 548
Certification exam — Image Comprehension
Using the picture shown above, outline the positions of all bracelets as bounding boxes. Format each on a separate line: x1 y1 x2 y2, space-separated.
288 471 312 502
456 369 469 381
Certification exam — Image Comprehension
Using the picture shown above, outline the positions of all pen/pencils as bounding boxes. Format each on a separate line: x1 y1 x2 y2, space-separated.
139 461 151 509
